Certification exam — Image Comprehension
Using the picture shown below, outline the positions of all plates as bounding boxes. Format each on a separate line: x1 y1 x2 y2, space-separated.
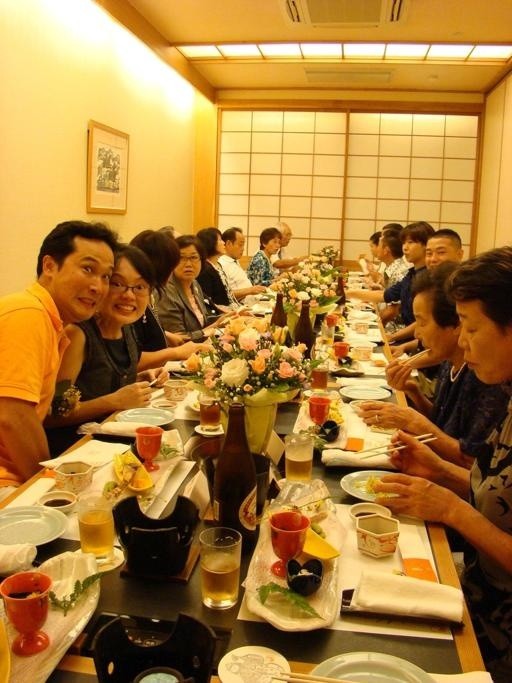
339 384 393 400
0 505 70 547
309 651 435 682
115 408 177 427
151 267 435 386
245 482 343 634
217 645 292 681
0 550 100 683
39 440 132 471
341 470 401 502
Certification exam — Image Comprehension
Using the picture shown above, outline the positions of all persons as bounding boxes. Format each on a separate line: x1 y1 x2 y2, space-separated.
343 220 512 683
0 217 306 505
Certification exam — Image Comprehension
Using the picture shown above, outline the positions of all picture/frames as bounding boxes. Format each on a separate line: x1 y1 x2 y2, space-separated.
85 119 130 216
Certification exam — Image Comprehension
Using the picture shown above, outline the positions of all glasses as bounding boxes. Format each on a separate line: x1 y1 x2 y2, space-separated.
179 253 202 263
108 280 154 297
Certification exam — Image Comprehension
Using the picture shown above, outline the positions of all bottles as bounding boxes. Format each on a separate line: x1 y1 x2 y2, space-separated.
210 402 258 560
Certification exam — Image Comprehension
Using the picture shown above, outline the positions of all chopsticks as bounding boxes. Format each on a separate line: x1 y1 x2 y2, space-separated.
354 432 438 460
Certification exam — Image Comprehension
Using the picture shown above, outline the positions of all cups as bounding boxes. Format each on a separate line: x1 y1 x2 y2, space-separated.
0 572 52 657
269 511 310 580
77 489 117 565
310 361 329 393
199 526 241 611
163 380 189 401
308 397 332 424
135 427 164 472
284 434 315 483
199 397 222 433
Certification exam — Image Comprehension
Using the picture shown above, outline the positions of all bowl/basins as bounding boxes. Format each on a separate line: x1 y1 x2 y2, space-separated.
158 429 185 459
52 461 95 496
152 400 179 413
38 490 78 515
349 399 384 412
356 513 400 560
348 501 394 522
